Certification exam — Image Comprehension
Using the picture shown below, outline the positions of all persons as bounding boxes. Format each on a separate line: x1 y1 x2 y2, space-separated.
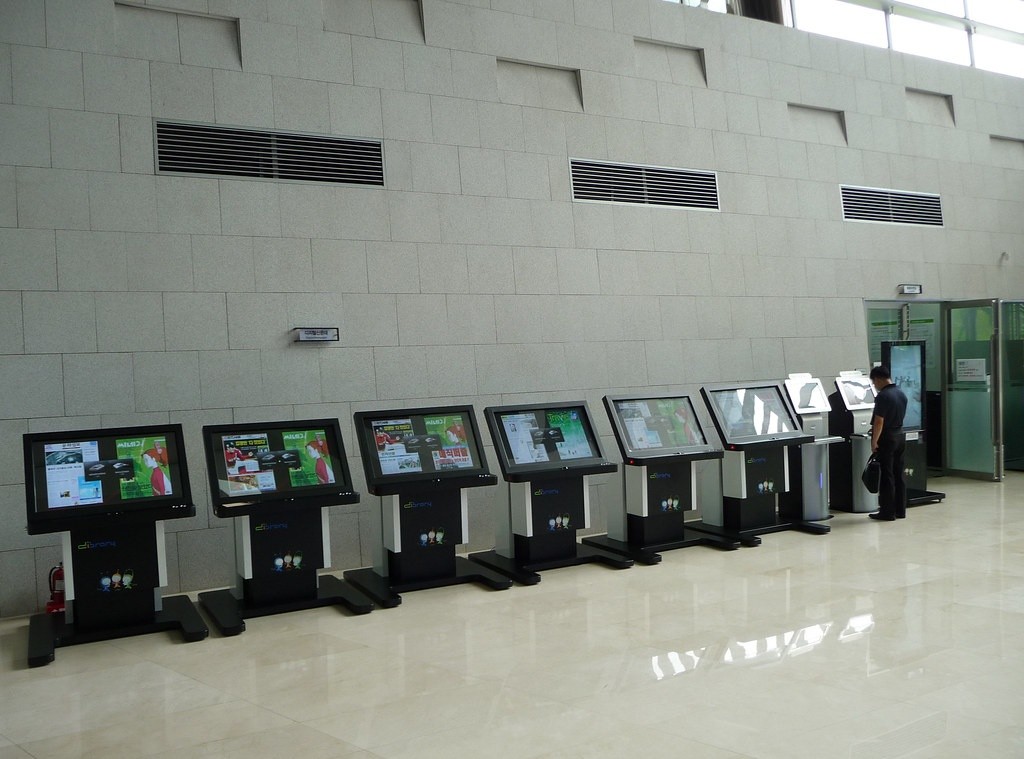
306 436 335 484
141 445 173 495
225 441 253 466
509 424 517 432
374 427 401 451
674 408 700 445
869 366 909 521
446 422 466 447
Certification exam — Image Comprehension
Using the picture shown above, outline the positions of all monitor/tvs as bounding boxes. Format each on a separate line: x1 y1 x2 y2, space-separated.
24 376 877 536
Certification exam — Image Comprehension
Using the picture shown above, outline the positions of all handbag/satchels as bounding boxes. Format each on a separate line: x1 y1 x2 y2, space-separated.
861 448 881 493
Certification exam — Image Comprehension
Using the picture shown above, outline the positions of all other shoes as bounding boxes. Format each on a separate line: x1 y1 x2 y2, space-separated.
869 511 905 521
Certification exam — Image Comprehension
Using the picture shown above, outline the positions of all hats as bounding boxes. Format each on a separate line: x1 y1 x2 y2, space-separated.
375 426 383 432
225 441 236 450
